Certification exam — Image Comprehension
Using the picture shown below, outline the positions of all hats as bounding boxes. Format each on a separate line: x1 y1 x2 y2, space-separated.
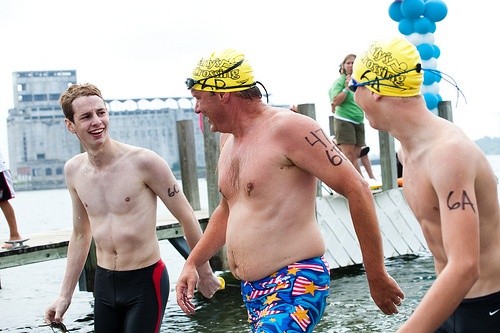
187 48 256 92
350 36 424 97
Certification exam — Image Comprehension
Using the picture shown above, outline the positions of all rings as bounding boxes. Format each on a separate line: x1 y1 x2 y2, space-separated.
394 298 401 305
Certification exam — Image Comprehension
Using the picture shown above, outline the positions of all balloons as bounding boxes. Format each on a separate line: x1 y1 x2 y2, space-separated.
388 0 447 111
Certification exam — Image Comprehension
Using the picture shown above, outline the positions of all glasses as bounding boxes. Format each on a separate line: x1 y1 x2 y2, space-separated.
50 322 70 333
348 63 421 93
185 58 244 90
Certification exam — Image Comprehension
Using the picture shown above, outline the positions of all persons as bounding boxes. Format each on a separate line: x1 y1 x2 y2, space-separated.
44 84 222 333
0 138 21 252
352 38 500 333
329 54 376 181
176 48 405 333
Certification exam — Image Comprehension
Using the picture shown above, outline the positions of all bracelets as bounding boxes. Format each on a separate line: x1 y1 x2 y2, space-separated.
342 89 349 94
